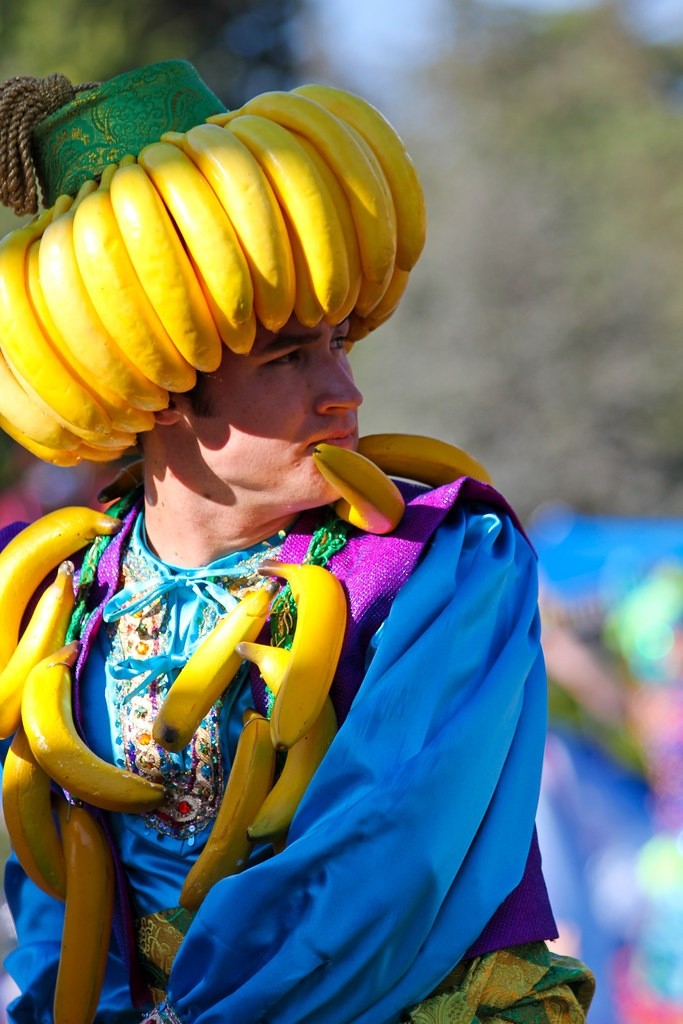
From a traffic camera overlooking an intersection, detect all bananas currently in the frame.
[0,81,427,467]
[0,433,495,1024]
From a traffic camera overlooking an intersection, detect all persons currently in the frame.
[525,570,683,1024]
[1,55,602,1024]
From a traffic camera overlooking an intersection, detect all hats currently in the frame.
[0,58,428,467]
